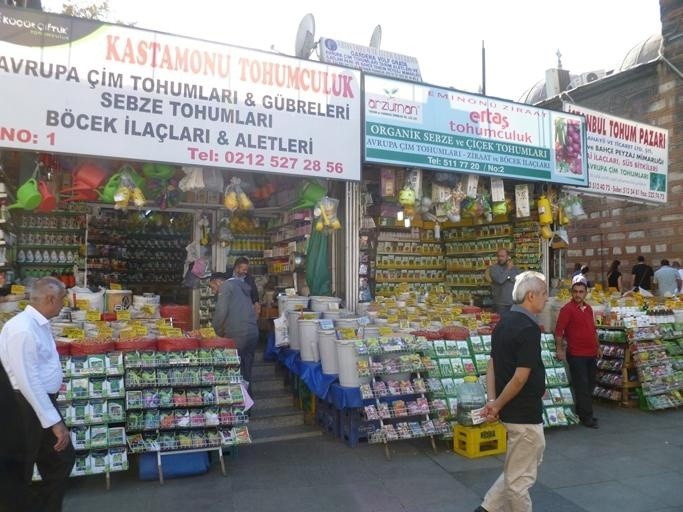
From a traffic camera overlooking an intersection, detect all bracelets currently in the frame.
[487,399,494,402]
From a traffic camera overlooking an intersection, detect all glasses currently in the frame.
[572,290,586,293]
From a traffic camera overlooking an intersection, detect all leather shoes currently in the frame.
[593,418,599,420]
[584,424,599,429]
[474,506,487,511]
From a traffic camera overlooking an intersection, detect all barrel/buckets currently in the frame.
[318,330,338,373]
[323,312,340,318]
[457,376,486,426]
[297,320,320,362]
[276,296,311,317]
[311,296,342,312]
[336,318,358,331]
[289,312,321,350]
[335,340,370,387]
[363,328,378,339]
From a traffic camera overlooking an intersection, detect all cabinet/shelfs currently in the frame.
[446,219,549,304]
[0,181,18,294]
[267,203,319,306]
[18,206,86,296]
[197,210,283,328]
[370,222,447,301]
[86,208,197,295]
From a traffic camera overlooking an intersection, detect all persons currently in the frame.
[654,260,682,299]
[572,267,590,288]
[475,272,548,511]
[569,264,583,278]
[211,274,259,415]
[490,250,517,316]
[607,261,622,295]
[0,278,75,511]
[632,256,653,298]
[555,282,601,427]
[671,261,682,299]
[229,257,261,322]
